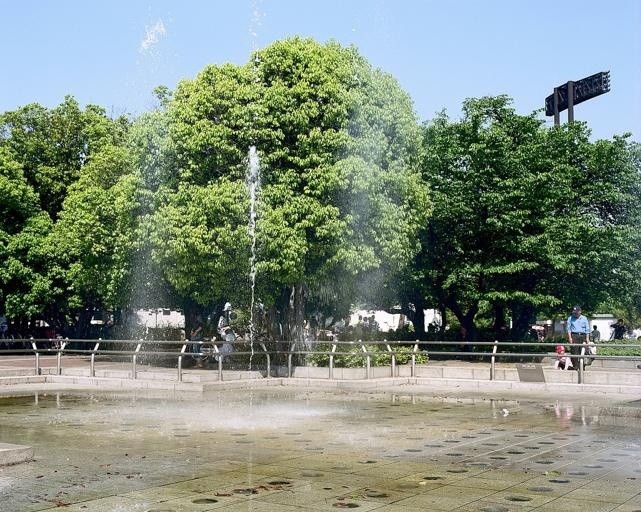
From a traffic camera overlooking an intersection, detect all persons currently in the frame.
[454,328,474,361]
[188,311,204,369]
[529,305,639,371]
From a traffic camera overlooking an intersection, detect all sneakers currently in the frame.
[568,366,578,370]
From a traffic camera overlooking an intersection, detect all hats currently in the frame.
[556,345,564,352]
[572,306,580,314]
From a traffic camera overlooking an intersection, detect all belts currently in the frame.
[572,332,585,334]
